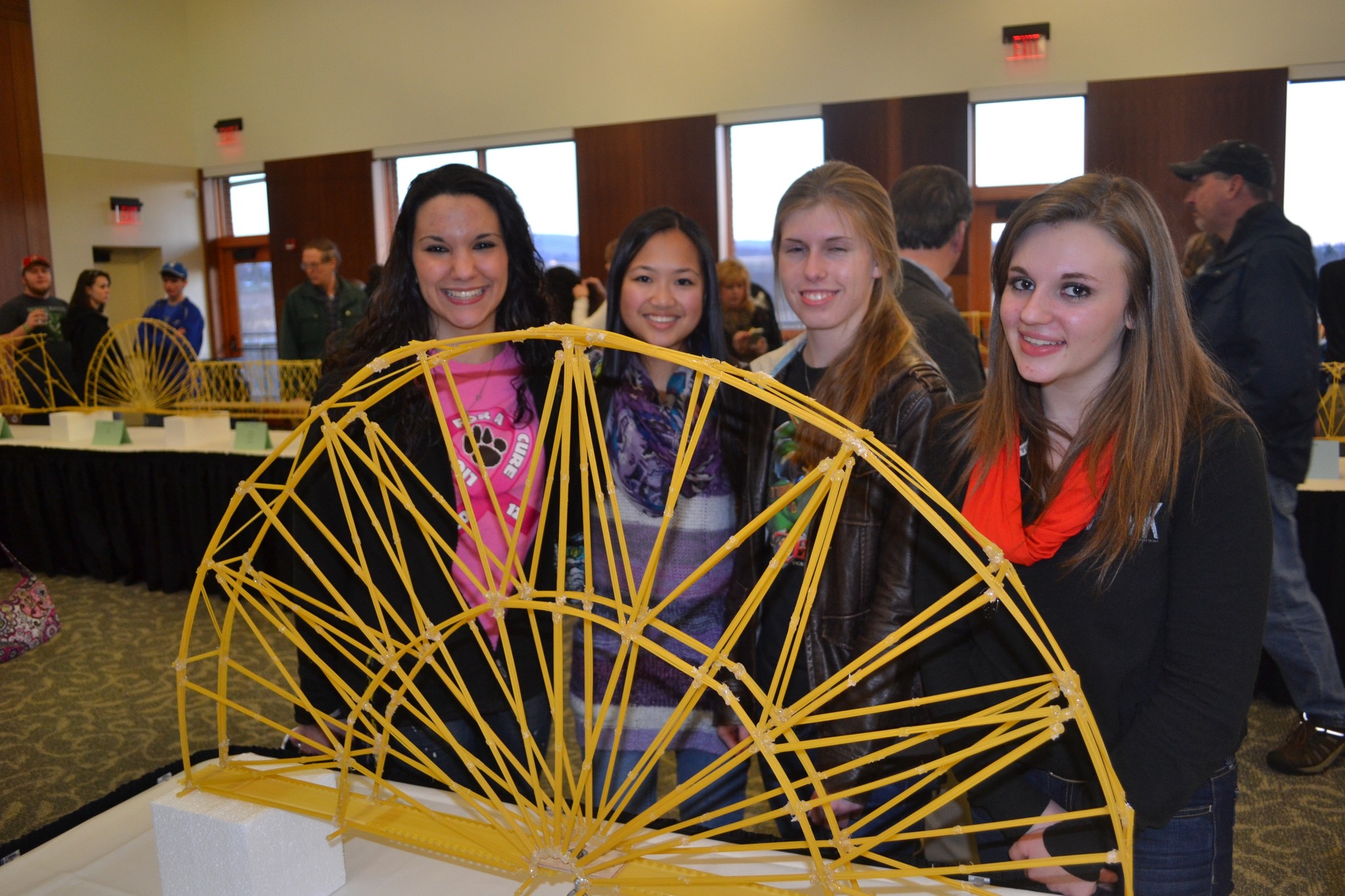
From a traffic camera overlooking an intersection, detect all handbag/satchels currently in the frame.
[0,542,61,665]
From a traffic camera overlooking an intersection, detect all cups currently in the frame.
[34,307,49,327]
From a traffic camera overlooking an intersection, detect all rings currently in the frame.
[37,320,42,324]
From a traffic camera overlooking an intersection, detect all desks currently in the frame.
[1,425,302,600]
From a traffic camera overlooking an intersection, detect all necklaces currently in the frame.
[1021,478,1046,502]
[465,346,498,414]
[804,347,814,395]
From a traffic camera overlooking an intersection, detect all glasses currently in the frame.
[300,260,329,270]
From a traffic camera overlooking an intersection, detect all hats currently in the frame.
[159,262,187,278]
[1168,139,1272,188]
[20,256,51,276]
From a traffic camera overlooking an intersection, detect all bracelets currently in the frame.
[21,322,29,335]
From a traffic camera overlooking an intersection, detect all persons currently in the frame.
[545,237,621,374]
[917,173,1273,896]
[1161,140,1345,776]
[132,259,205,406]
[285,237,385,411]
[888,165,987,410]
[712,259,782,367]
[711,161,958,867]
[284,164,600,838]
[556,206,754,838]
[0,255,69,425]
[58,269,128,410]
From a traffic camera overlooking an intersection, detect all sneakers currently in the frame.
[1269,714,1345,773]
